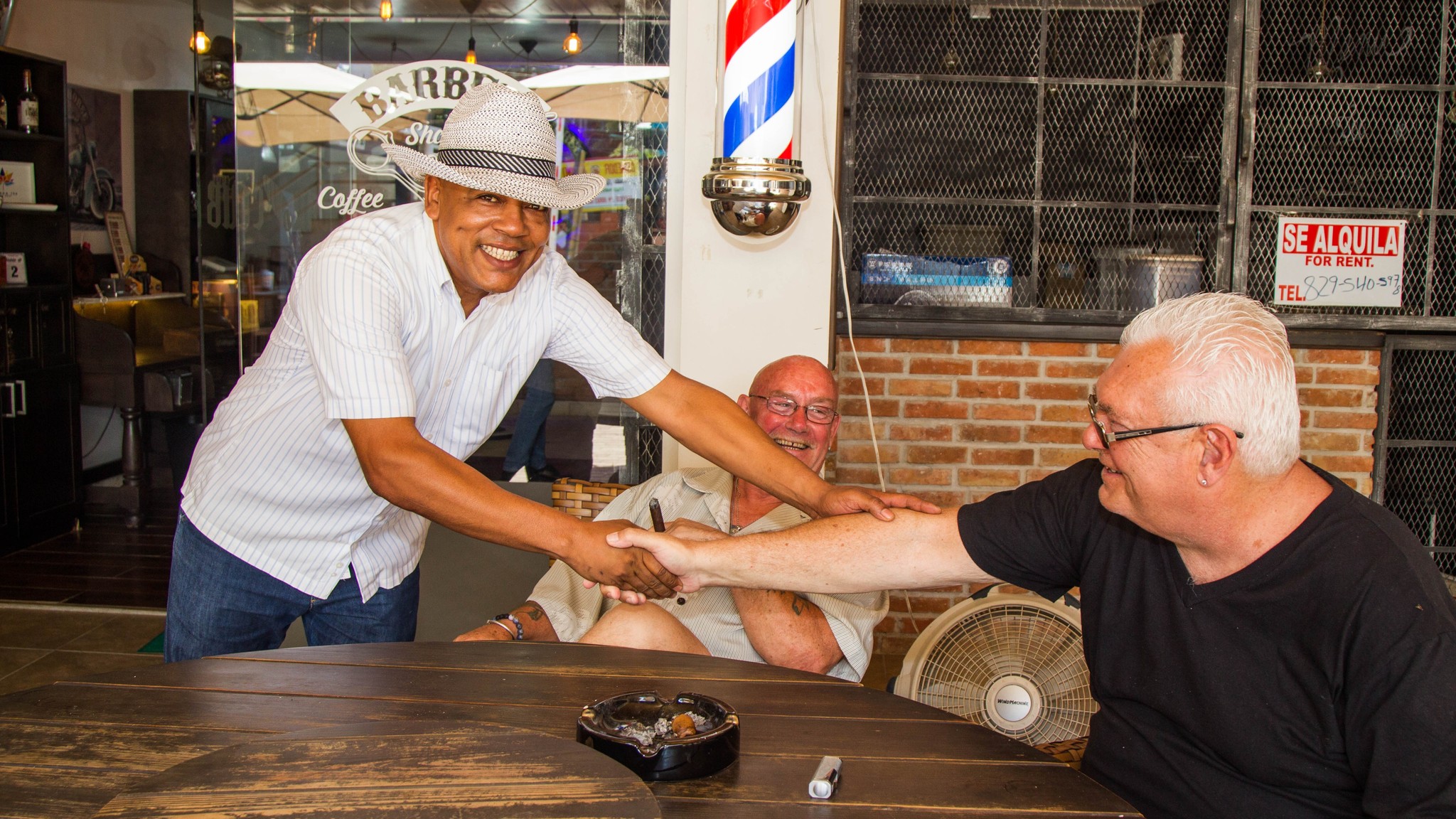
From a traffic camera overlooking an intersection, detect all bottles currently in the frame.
[0,93,7,129]
[17,69,38,132]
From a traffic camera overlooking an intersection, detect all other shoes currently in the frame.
[529,463,567,483]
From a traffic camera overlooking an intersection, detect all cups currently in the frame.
[258,272,274,291]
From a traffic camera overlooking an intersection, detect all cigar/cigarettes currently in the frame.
[649,498,665,533]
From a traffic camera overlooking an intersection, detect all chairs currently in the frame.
[551,474,632,564]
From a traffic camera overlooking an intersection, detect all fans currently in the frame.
[894,593,1096,748]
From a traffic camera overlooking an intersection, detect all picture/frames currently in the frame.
[0,161,36,204]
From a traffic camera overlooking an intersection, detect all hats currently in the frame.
[380,83,605,210]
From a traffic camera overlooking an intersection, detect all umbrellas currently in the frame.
[236,59,676,149]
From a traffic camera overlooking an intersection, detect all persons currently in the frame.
[455,355,889,683]
[581,294,1456,818]
[165,87,943,662]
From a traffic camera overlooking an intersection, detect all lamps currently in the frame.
[702,0,811,238]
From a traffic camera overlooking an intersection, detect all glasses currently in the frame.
[1087,393,1243,450]
[749,394,838,424]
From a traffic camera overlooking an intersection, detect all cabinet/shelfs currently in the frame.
[0,46,76,561]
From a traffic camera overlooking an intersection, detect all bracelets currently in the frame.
[487,612,522,641]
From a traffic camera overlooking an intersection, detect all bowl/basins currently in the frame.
[924,224,1005,258]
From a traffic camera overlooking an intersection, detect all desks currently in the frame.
[0,643,1148,819]
[73,289,238,527]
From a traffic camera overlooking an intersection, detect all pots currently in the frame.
[1095,254,1205,312]
